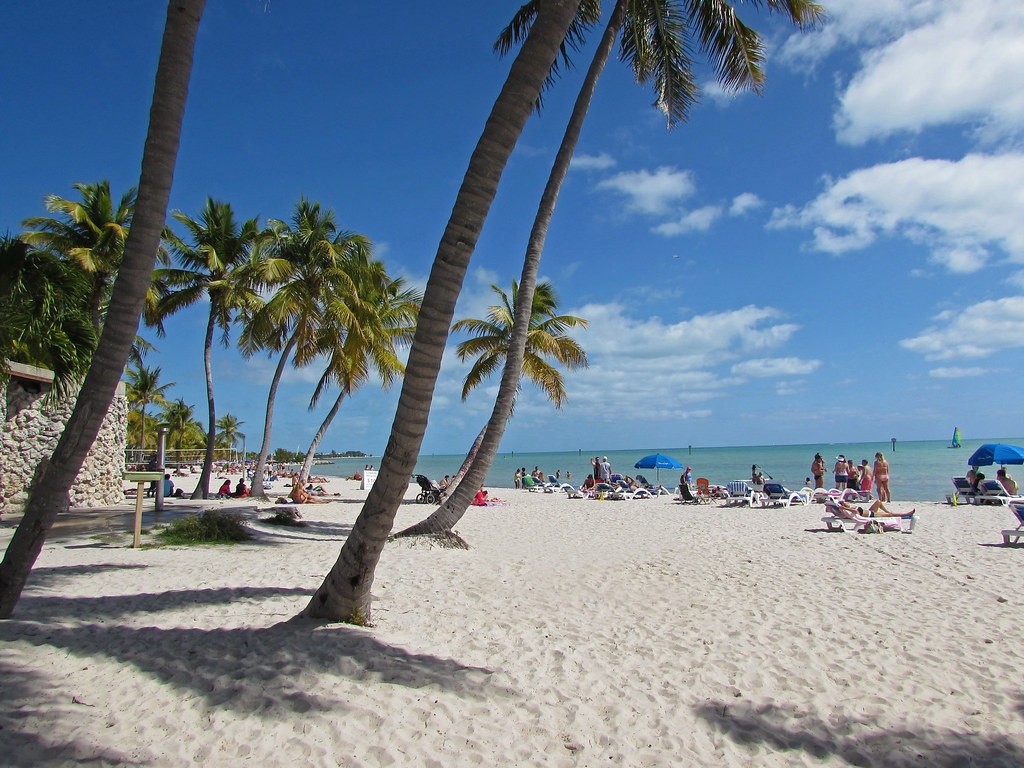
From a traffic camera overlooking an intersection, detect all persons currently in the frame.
[582,456,661,491]
[996,470,1017,495]
[811,453,825,488]
[806,477,811,485]
[838,500,915,517]
[355,464,374,480]
[440,474,455,488]
[566,471,572,479]
[967,466,985,495]
[682,466,692,485]
[513,466,544,489]
[164,474,174,497]
[832,451,891,502]
[471,484,489,506]
[752,465,765,484]
[189,464,329,504]
[556,470,561,478]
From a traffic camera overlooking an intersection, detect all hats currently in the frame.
[686,466,692,470]
[603,456,608,461]
[835,455,845,462]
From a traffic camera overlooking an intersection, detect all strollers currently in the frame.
[411,473,440,506]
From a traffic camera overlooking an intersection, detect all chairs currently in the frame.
[522,474,920,534]
[945,476,1024,544]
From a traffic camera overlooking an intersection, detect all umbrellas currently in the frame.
[634,452,682,481]
[968,443,1024,469]
